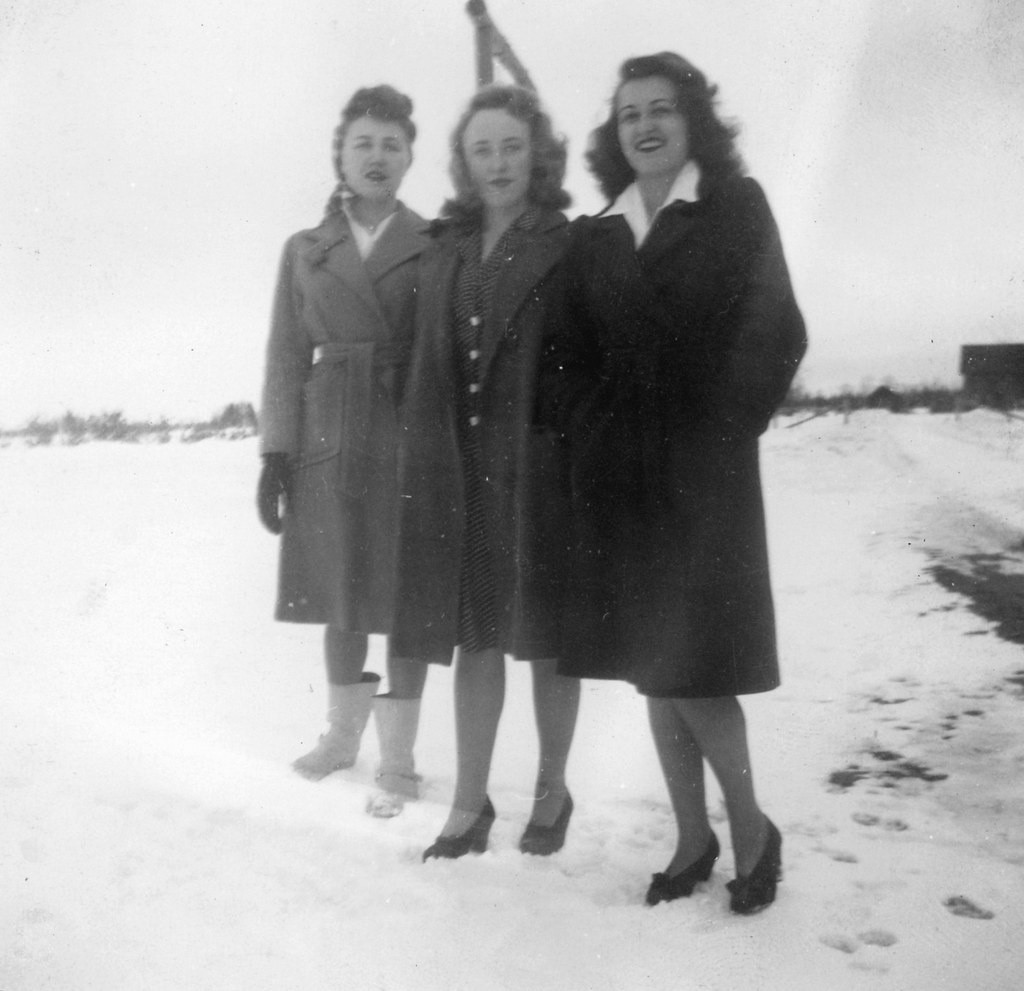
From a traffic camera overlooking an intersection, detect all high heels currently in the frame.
[646,837,719,911]
[426,796,494,863]
[516,798,577,855]
[727,820,782,915]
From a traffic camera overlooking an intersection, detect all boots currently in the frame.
[293,674,378,779]
[368,694,421,818]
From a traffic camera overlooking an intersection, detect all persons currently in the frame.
[388,82,576,863]
[539,50,810,915]
[255,75,438,811]
